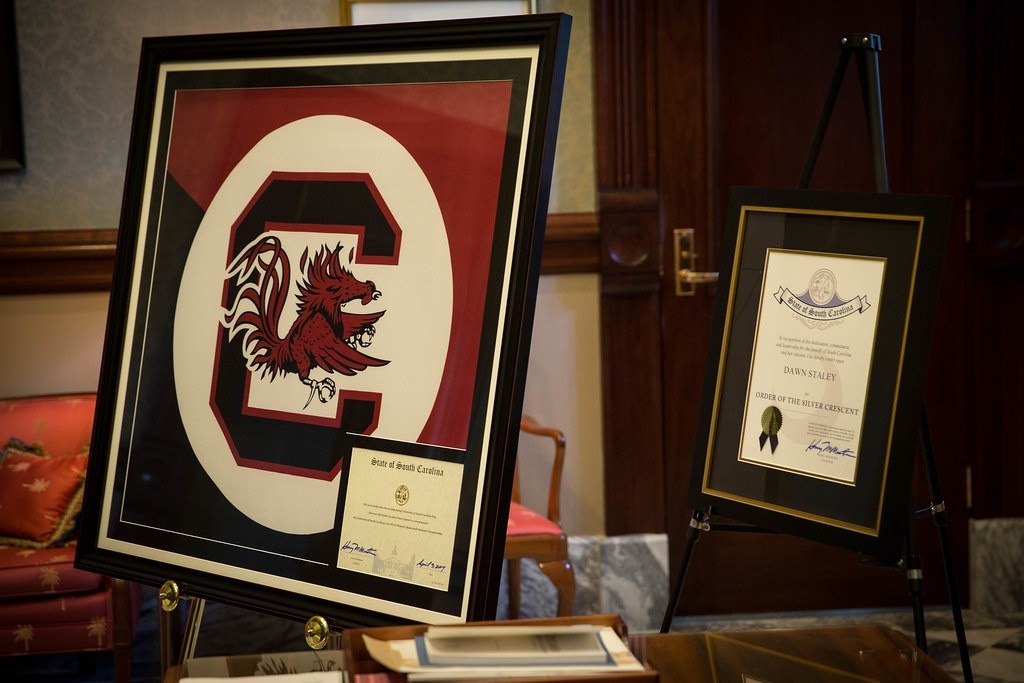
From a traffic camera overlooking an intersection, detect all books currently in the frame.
[364,622,648,683]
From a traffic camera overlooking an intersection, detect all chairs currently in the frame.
[493,420,570,621]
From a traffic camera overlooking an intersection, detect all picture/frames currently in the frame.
[73,12,571,624]
[702,207,925,541]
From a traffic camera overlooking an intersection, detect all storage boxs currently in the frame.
[968,520,1023,611]
[495,533,670,633]
[339,612,659,683]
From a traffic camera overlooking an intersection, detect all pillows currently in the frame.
[0,398,96,549]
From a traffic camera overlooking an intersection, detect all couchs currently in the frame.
[0,387,146,683]
